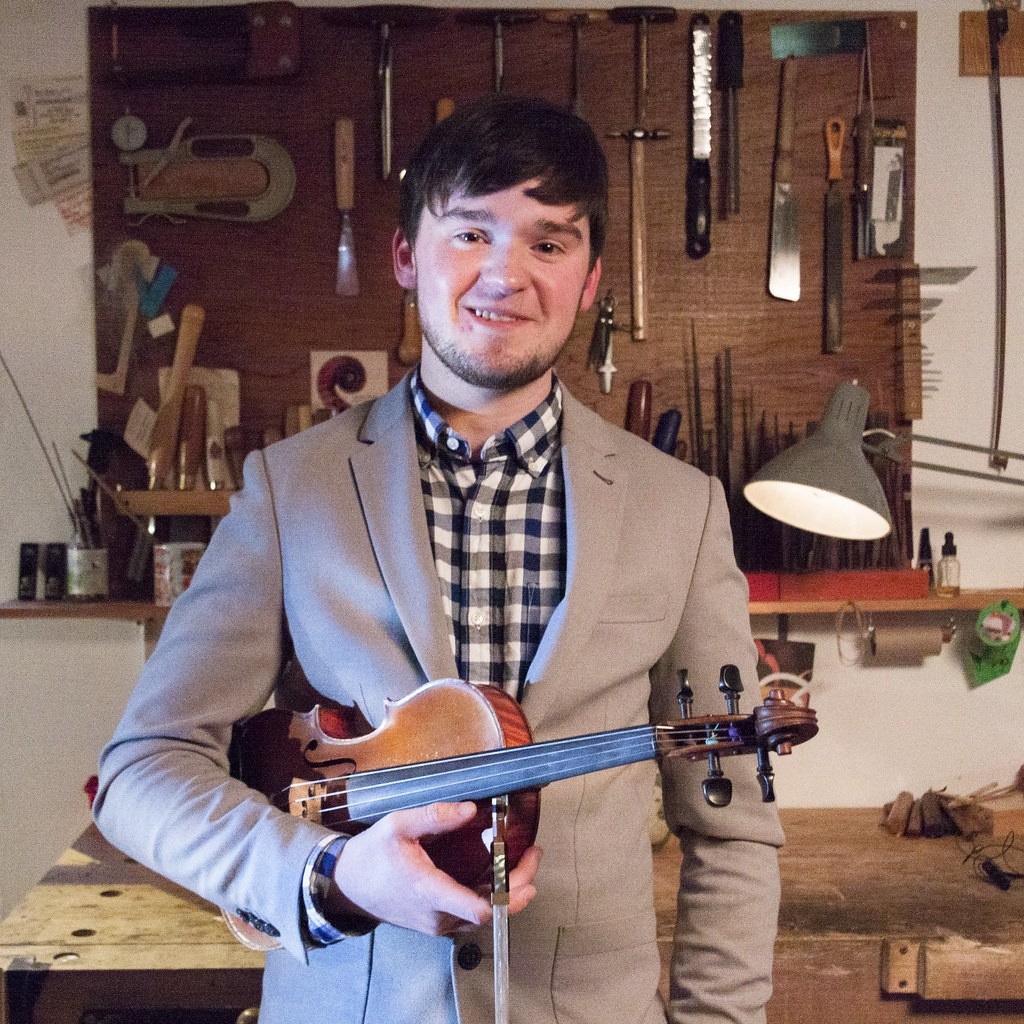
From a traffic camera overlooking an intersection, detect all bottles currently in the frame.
[936,532,960,598]
[67,499,109,602]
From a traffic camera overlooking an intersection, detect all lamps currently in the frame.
[742,378,1024,540]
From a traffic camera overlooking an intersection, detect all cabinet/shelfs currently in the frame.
[2,487,1024,663]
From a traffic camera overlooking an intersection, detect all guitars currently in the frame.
[222,661,820,951]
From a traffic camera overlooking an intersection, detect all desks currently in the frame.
[0,801,1024,1024]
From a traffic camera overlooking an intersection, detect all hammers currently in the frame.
[608,5,679,121]
[607,128,671,345]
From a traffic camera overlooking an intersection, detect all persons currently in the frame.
[88,92,798,1024]
